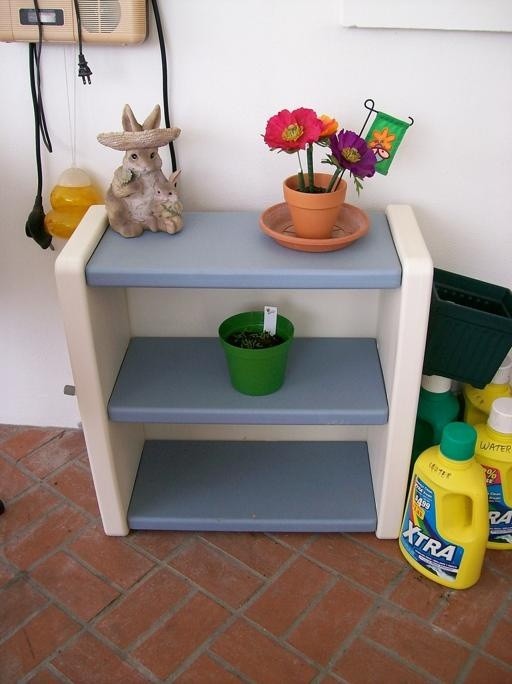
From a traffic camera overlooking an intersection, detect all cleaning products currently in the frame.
[476,397,512,553]
[413,374,460,462]
[462,358,511,424]
[394,419,491,591]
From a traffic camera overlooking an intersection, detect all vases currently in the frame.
[217,310,294,398]
[282,172,348,239]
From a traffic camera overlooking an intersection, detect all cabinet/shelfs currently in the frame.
[52,203,435,540]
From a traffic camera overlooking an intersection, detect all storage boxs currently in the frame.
[423,267,511,390]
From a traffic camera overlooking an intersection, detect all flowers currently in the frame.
[258,105,378,199]
